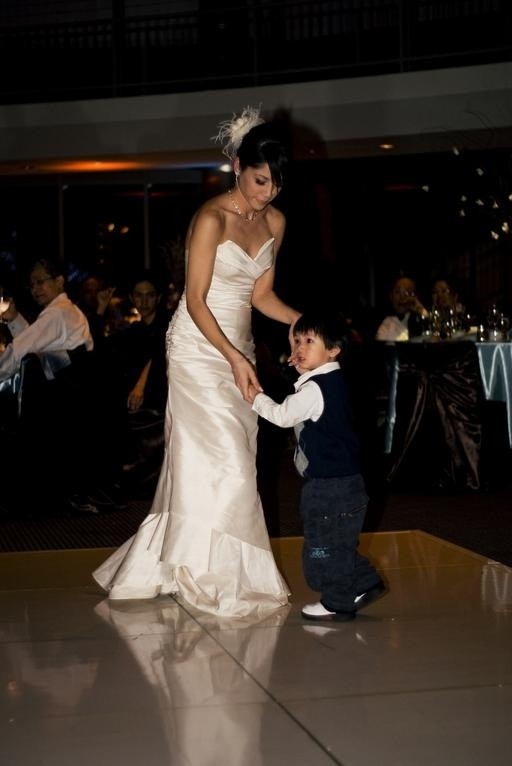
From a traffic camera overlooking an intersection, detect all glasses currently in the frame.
[28,276,59,289]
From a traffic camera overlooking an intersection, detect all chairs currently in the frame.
[391,340,495,498]
[17,353,48,418]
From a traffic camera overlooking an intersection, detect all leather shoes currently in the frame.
[300,600,357,623]
[356,582,384,609]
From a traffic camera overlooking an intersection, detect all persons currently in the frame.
[371,270,476,341]
[246,308,390,620]
[81,268,218,472]
[0,255,96,438]
[93,105,305,618]
